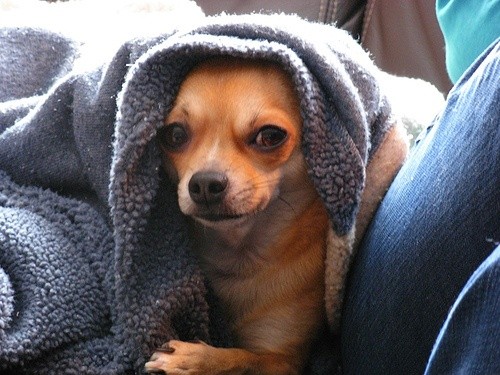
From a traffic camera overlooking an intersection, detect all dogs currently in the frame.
[143,59,408,375]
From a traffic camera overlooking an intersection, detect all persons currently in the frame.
[336,1,500,375]
